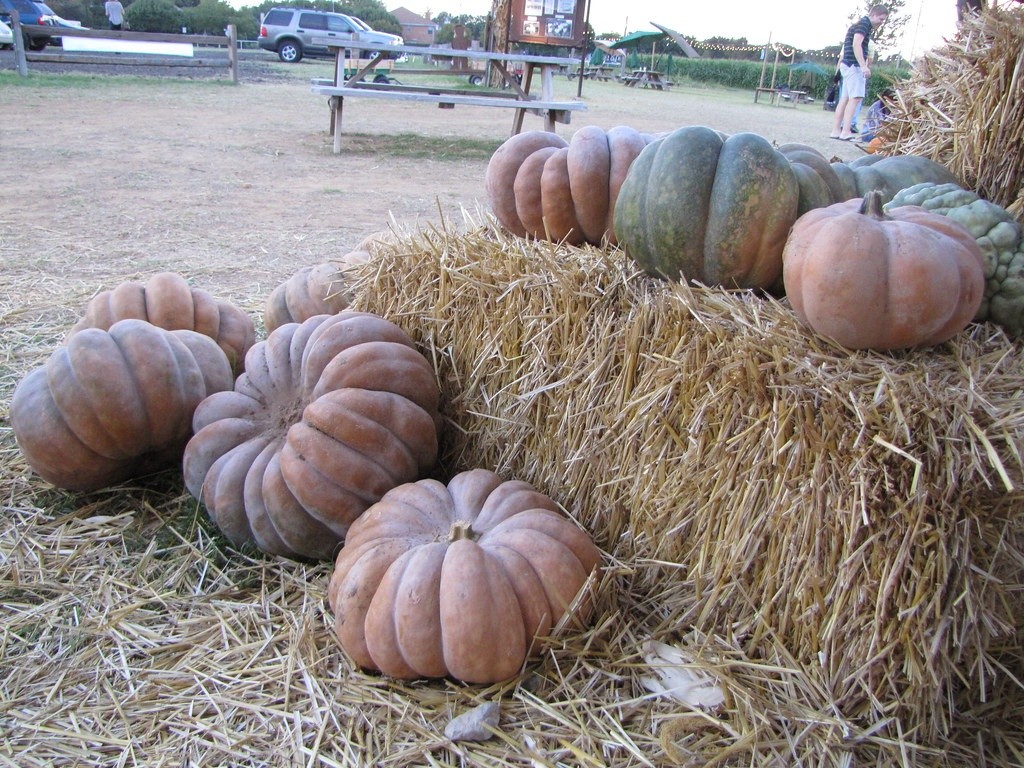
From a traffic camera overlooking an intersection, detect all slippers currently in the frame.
[830,135,840,139]
[838,134,855,141]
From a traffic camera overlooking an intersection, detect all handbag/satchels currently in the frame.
[823,83,839,112]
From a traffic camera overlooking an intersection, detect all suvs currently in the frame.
[257,5,404,63]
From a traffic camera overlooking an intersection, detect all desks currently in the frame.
[311,37,582,154]
[572,67,614,82]
[624,70,671,92]
[754,87,799,109]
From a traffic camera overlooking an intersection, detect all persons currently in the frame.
[823,4,898,154]
[104,0,125,55]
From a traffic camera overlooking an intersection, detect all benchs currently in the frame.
[309,77,586,110]
[570,72,614,79]
[621,76,674,86]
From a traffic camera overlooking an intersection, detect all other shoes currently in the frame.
[850,125,860,132]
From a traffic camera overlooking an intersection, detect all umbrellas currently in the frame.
[589,21,700,88]
[786,61,830,85]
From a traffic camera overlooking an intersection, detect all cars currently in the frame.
[0,0,58,51]
[351,16,374,31]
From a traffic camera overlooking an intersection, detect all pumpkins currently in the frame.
[329,469,605,684]
[484,122,1024,352]
[7,319,235,491]
[59,272,255,381]
[263,233,400,336]
[183,310,439,559]
